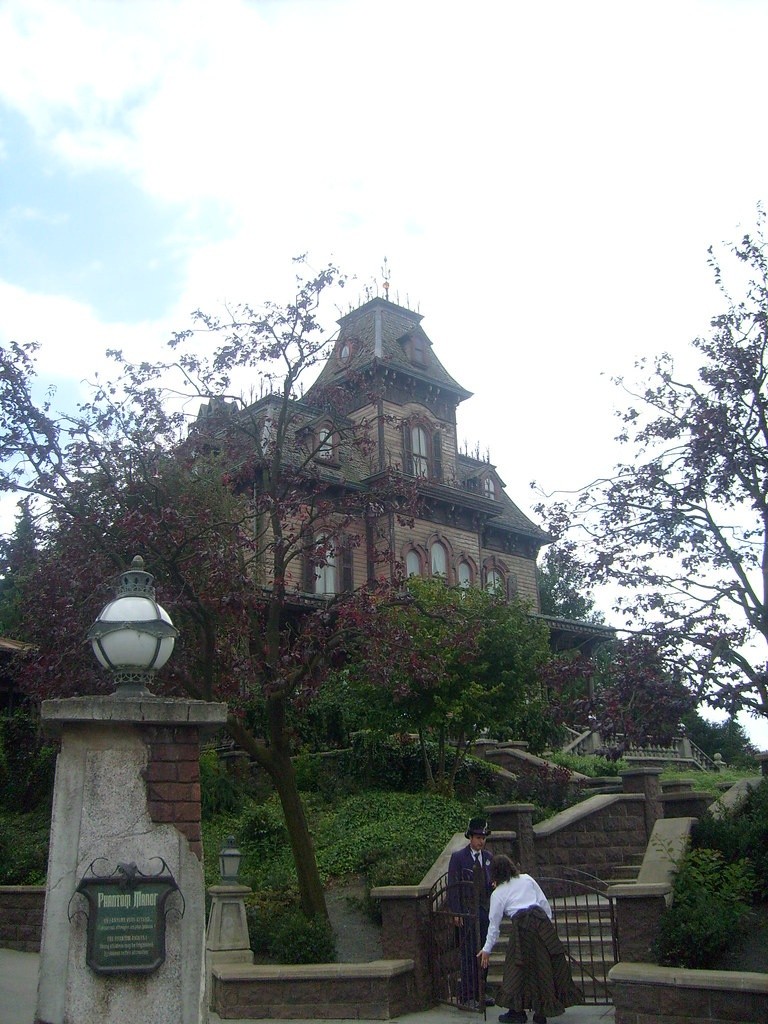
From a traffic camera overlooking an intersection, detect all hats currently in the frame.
[464,817,491,839]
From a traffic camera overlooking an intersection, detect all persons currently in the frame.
[447,818,499,1009]
[476,853,583,1024]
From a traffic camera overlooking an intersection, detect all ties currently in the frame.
[469,848,489,912]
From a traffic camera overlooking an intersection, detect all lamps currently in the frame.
[81,555,181,698]
[676,721,686,736]
[215,835,244,884]
[473,722,491,739]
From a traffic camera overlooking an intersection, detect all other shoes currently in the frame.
[533,1013,547,1023]
[458,1000,480,1011]
[498,1009,528,1024]
[478,995,495,1006]
